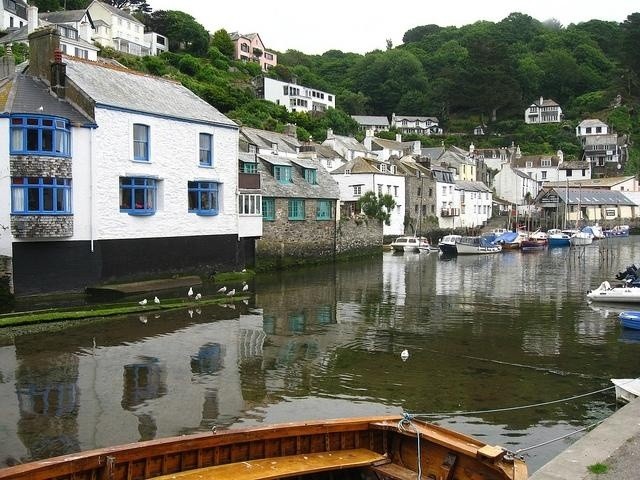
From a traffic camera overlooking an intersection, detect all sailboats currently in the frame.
[438,173,605,261]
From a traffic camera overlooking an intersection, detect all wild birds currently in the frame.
[154,296,160,304]
[242,284,248,293]
[216,286,226,294]
[188,287,194,297]
[227,288,236,298]
[400,349,409,361]
[138,299,147,306]
[195,293,201,301]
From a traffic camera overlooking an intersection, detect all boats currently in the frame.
[585,301,640,319]
[583,280,640,304]
[2,408,534,480]
[615,310,640,331]
[388,234,432,253]
[392,252,421,265]
[603,224,630,238]
[418,247,441,254]
